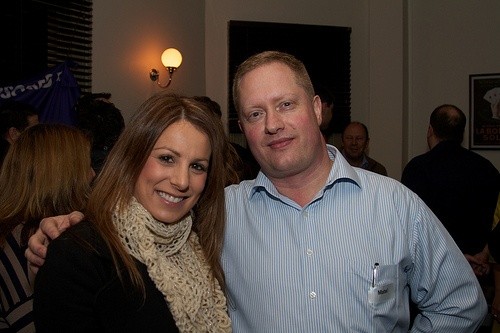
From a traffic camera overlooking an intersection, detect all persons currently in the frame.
[24,50,488,333]
[33,94,237,333]
[312,74,388,178]
[401,105,500,333]
[0,105,95,333]
[193,96,261,183]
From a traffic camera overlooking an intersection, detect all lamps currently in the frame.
[150,48,182,89]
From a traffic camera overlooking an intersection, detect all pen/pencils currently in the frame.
[372,263,380,288]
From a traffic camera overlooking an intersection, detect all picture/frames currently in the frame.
[469,73,500,151]
